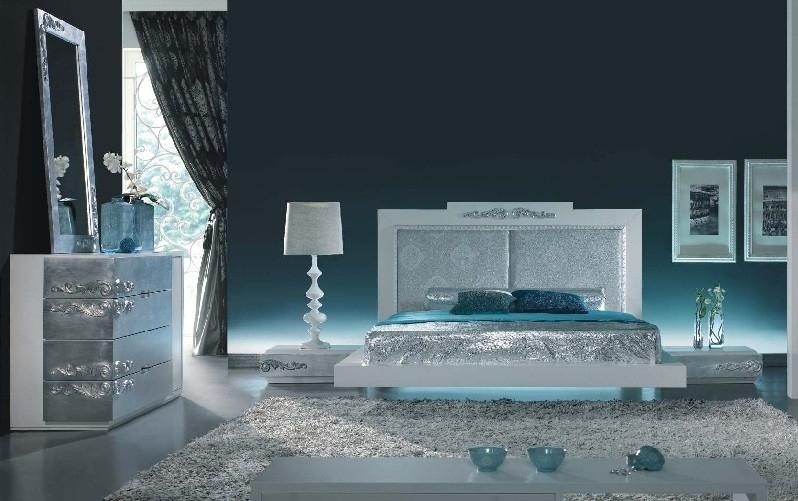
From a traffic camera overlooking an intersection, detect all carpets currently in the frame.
[98,396,798,501]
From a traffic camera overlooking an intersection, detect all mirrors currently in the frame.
[34,7,99,254]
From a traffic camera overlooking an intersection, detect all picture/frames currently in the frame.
[671,159,737,264]
[744,158,789,262]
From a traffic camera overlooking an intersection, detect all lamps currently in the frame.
[283,201,344,349]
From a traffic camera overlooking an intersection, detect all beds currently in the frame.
[335,286,688,408]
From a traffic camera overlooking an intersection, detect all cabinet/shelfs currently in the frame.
[8,252,186,431]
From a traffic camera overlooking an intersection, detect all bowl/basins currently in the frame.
[469,446,508,472]
[527,446,565,472]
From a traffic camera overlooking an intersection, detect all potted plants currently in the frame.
[693,286,712,350]
[708,282,728,350]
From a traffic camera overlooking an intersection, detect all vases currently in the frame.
[130,198,156,252]
[102,197,136,253]
[59,201,77,236]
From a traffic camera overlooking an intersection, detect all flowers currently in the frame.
[53,153,71,203]
[104,152,176,211]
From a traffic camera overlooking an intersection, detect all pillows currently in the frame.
[513,291,586,298]
[452,289,503,308]
